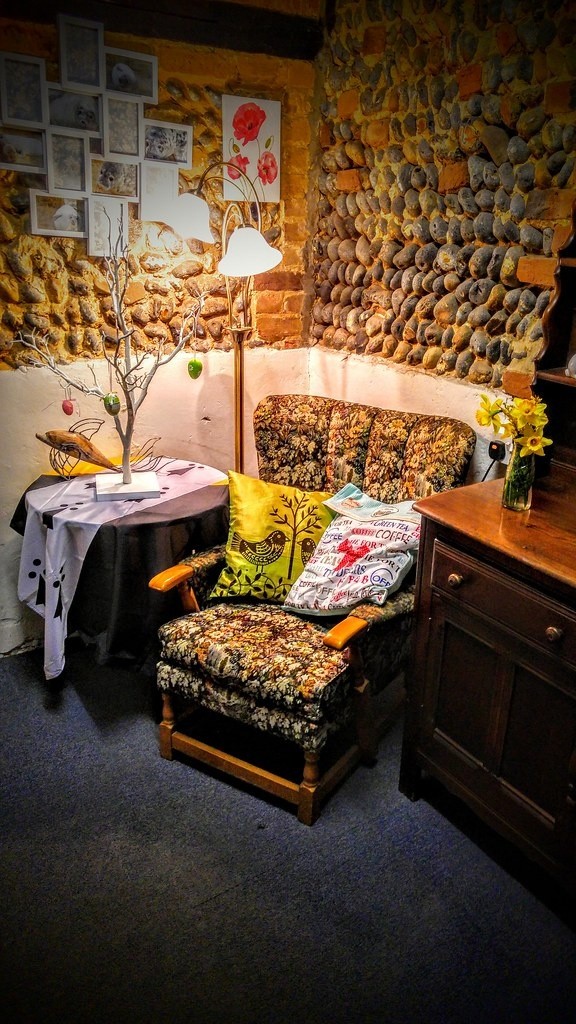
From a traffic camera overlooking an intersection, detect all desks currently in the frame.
[10,458,228,680]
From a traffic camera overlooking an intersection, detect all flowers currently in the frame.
[477,395,553,457]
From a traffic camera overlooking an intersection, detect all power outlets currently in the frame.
[499,443,513,465]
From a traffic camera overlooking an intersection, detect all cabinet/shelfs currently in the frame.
[396,193,576,922]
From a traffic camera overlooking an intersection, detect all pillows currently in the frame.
[283,483,421,616]
[209,470,335,601]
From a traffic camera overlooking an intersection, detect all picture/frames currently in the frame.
[0,15,192,256]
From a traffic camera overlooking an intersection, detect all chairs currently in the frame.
[148,394,476,826]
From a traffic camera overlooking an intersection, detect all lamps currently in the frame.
[152,162,283,475]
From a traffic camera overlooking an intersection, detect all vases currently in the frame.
[502,440,537,511]
